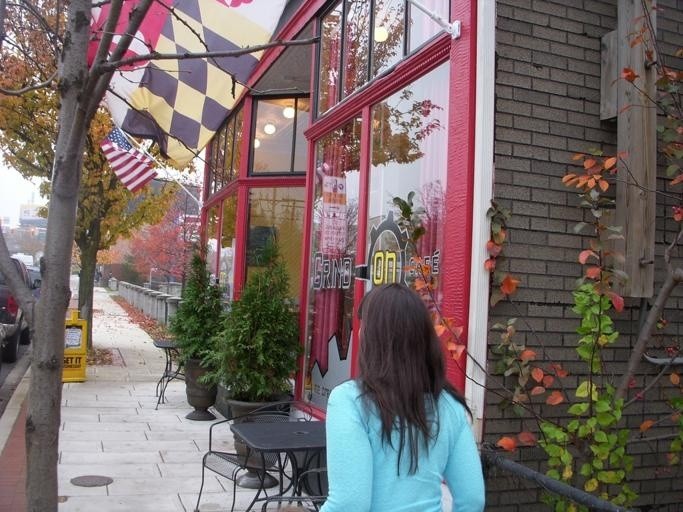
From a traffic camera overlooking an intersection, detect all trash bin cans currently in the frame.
[111,279,118,291]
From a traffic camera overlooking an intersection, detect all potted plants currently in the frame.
[166,223,302,428]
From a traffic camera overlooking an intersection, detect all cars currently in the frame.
[0,254,39,365]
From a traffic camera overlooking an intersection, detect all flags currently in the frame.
[98,127,158,193]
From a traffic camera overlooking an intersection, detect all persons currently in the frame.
[311,283,487,510]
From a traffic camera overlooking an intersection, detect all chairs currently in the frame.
[150,338,192,412]
[193,400,328,511]
